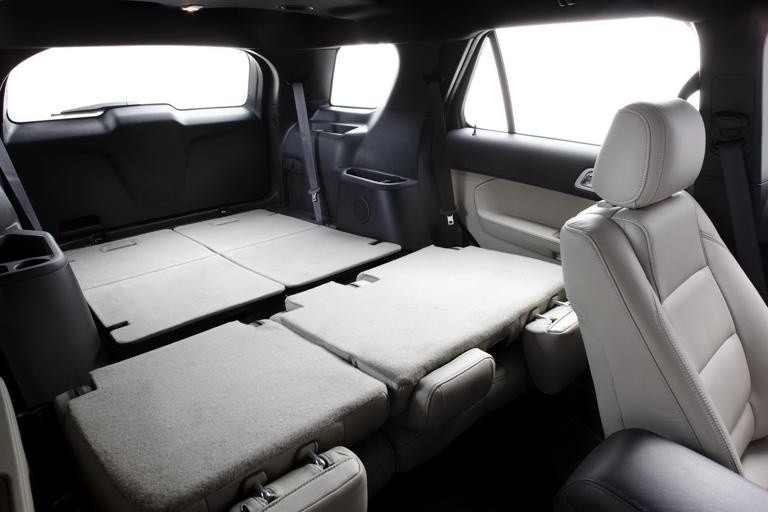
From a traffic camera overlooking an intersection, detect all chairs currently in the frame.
[559,98,768,512]
